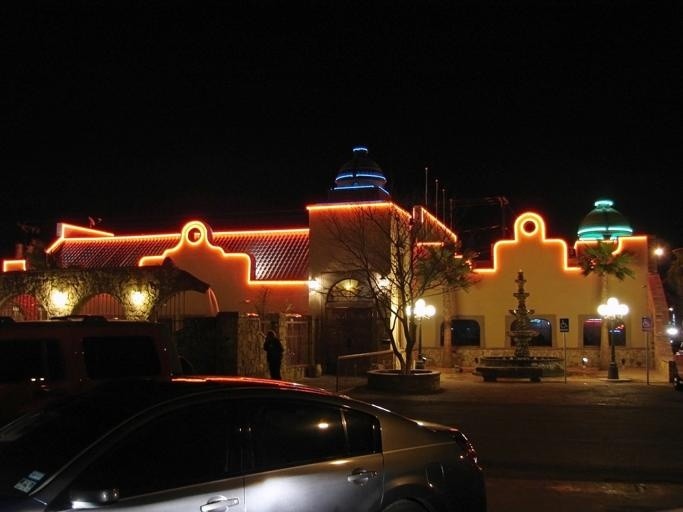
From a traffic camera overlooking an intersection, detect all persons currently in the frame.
[261,330,286,379]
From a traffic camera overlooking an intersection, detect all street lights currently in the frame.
[406,300,437,371]
[598,297,631,380]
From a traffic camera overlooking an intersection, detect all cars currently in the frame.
[1,375,488,512]
[674,340,683,392]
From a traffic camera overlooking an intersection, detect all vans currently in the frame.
[0,317,180,419]
